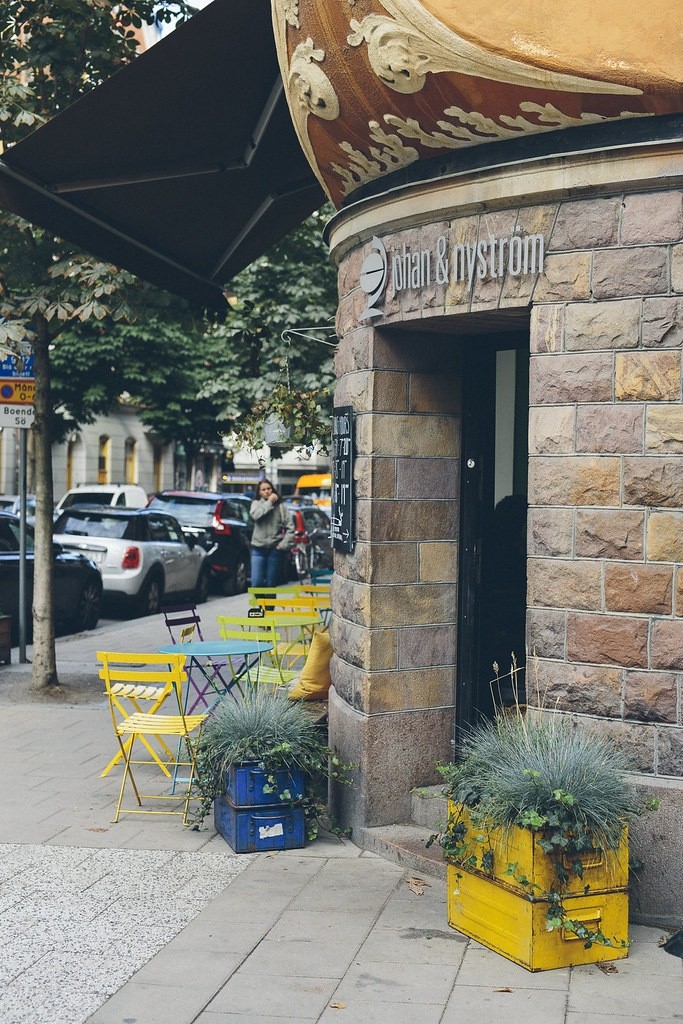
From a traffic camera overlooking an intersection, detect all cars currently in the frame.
[57,481,149,518]
[0,493,48,526]
[280,473,331,540]
[49,505,206,617]
[0,510,104,653]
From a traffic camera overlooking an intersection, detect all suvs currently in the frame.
[142,490,264,592]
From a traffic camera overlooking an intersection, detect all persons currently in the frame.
[247,478,296,611]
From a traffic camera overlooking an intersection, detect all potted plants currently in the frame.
[184,696,359,855]
[434,703,638,976]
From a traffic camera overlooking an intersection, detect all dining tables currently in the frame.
[156,639,276,781]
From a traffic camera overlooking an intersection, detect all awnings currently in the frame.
[0,0,330,310]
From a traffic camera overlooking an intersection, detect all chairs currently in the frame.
[217,569,331,698]
[93,650,207,826]
[162,602,217,676]
